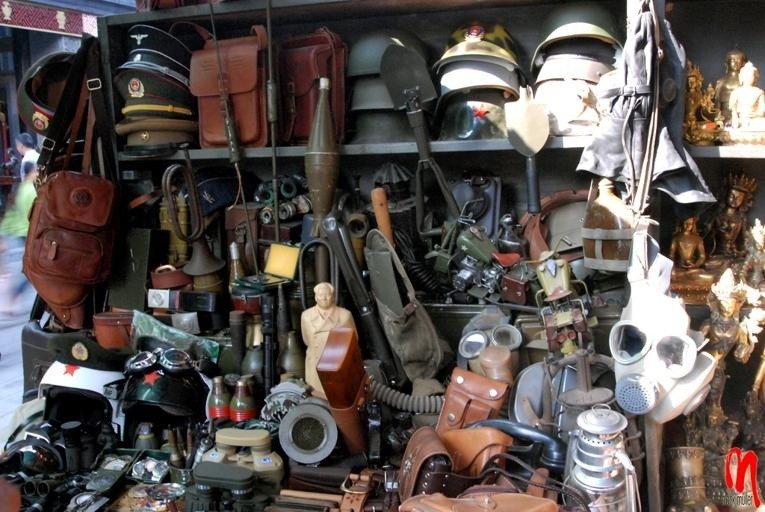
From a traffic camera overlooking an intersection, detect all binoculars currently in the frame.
[24,472,67,498]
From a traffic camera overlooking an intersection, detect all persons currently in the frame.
[665,171,765,489]
[688,46,765,132]
[0,133,40,308]
[300,282,359,400]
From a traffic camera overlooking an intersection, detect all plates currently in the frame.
[279,404,338,464]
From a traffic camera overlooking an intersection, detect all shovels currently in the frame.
[380,45,460,237]
[504,101,550,213]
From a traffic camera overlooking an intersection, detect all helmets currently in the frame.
[348,3,622,142]
[1,349,214,473]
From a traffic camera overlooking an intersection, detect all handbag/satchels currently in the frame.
[400,372,560,511]
[193,30,346,149]
[22,170,117,402]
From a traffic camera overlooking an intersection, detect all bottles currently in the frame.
[205,310,303,424]
[224,242,245,293]
[136,419,156,451]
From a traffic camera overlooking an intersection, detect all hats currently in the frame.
[23,25,196,156]
